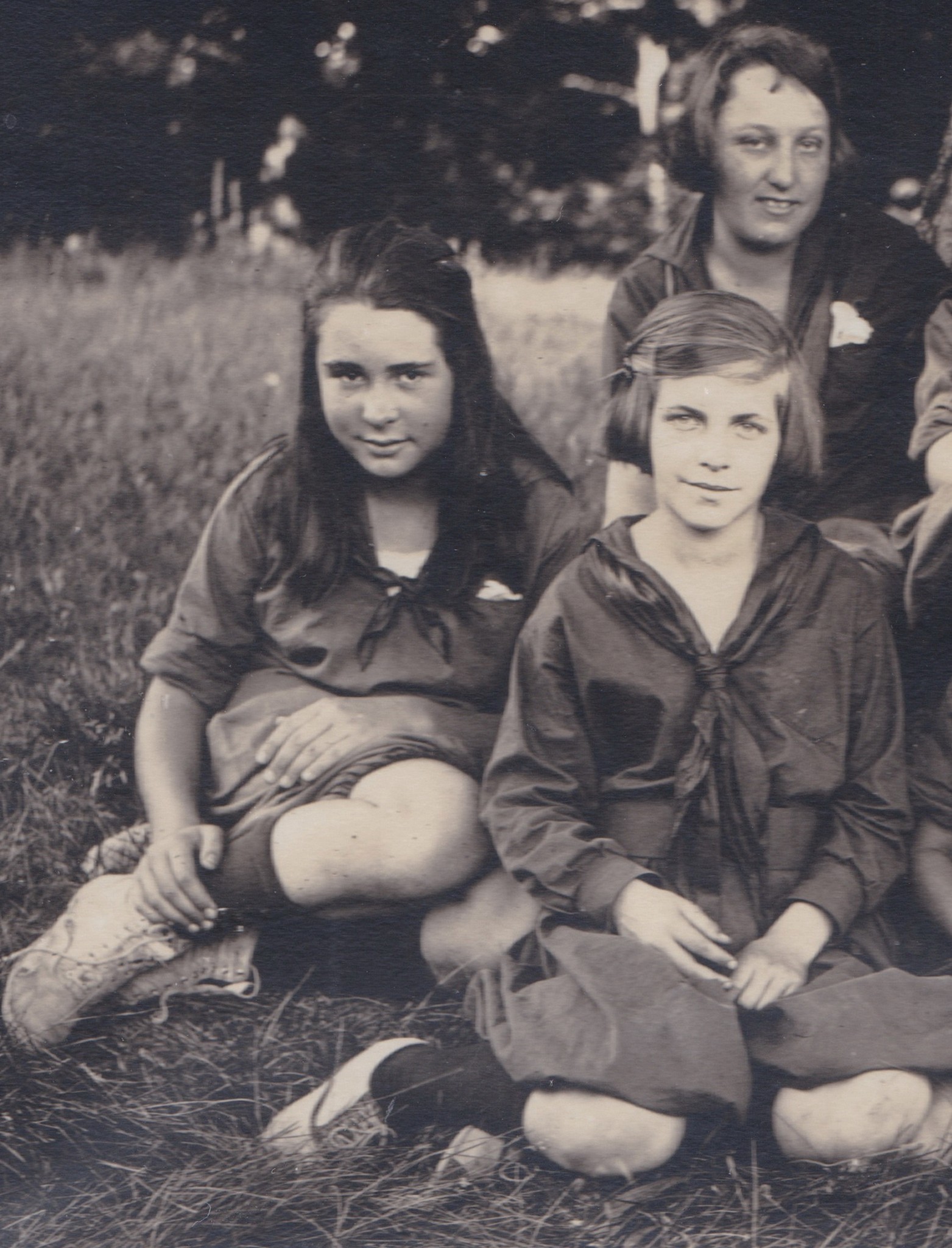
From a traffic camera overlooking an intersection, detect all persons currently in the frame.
[910,754,952,936]
[907,299,952,489]
[598,22,952,618]
[1,219,598,1054]
[259,290,935,1183]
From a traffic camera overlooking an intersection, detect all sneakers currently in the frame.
[429,1124,532,1183]
[258,1038,436,1167]
[2,873,196,1056]
[106,919,262,1002]
